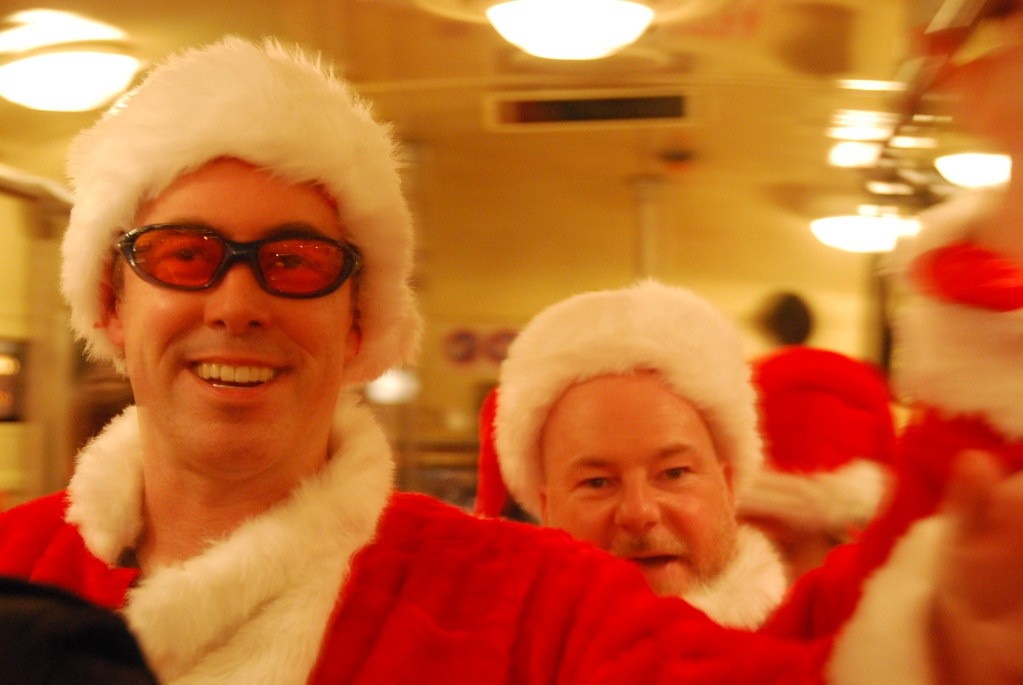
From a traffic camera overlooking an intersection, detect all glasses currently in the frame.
[113,222,363,299]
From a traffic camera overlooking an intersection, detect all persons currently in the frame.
[752,241,1022,635]
[496,290,805,636]
[0,38,1023,685]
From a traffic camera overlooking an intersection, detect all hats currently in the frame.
[889,242,1023,441]
[491,279,764,523]
[60,37,423,386]
[737,347,894,535]
